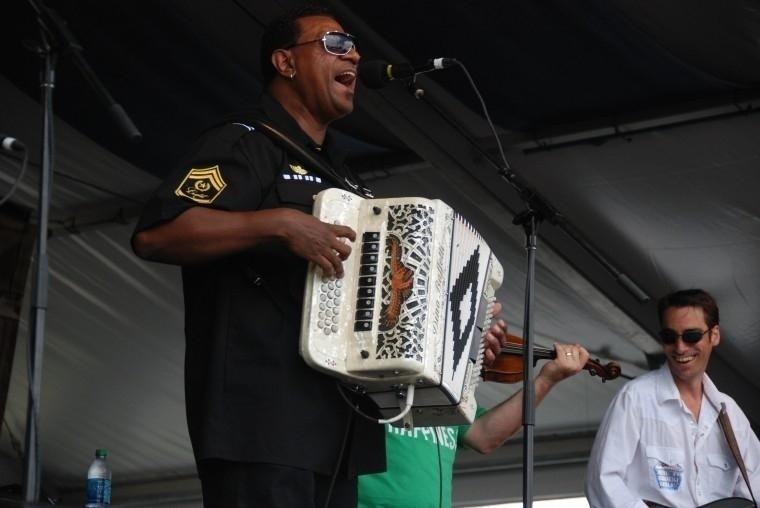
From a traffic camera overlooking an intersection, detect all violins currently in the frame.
[480,335,621,383]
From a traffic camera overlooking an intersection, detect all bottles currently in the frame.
[84,447,113,508]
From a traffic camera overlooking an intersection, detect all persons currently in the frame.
[358,341,591,506]
[132,9,510,507]
[584,288,760,505]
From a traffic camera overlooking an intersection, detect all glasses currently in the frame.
[291,32,358,55]
[660,326,709,342]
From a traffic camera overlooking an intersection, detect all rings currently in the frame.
[565,351,573,356]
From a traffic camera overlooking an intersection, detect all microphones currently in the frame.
[358,57,454,92]
